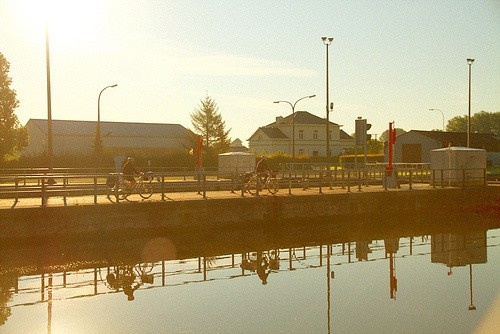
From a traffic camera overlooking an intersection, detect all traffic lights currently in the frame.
[355,120,374,148]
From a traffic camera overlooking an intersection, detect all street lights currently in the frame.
[95,84,117,143]
[467,265,476,311]
[321,36,335,176]
[429,107,446,130]
[272,94,317,164]
[464,58,475,148]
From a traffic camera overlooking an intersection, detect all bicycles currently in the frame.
[247,249,280,272]
[113,262,155,289]
[246,169,280,195]
[114,173,154,200]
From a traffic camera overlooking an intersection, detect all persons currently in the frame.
[122,157,145,193]
[256,156,271,189]
